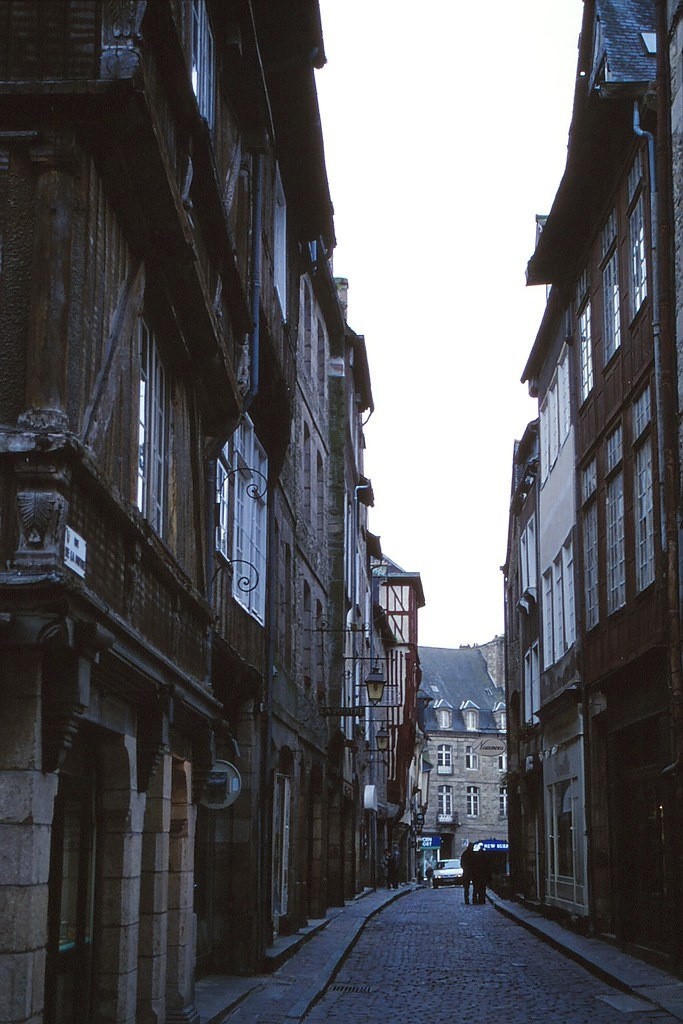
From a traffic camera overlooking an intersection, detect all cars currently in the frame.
[433,859,465,889]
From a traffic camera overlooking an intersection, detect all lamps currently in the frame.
[360,720,393,753]
[330,656,389,707]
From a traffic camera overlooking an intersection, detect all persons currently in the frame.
[416,862,433,884]
[460,842,491,904]
[380,843,400,889]
